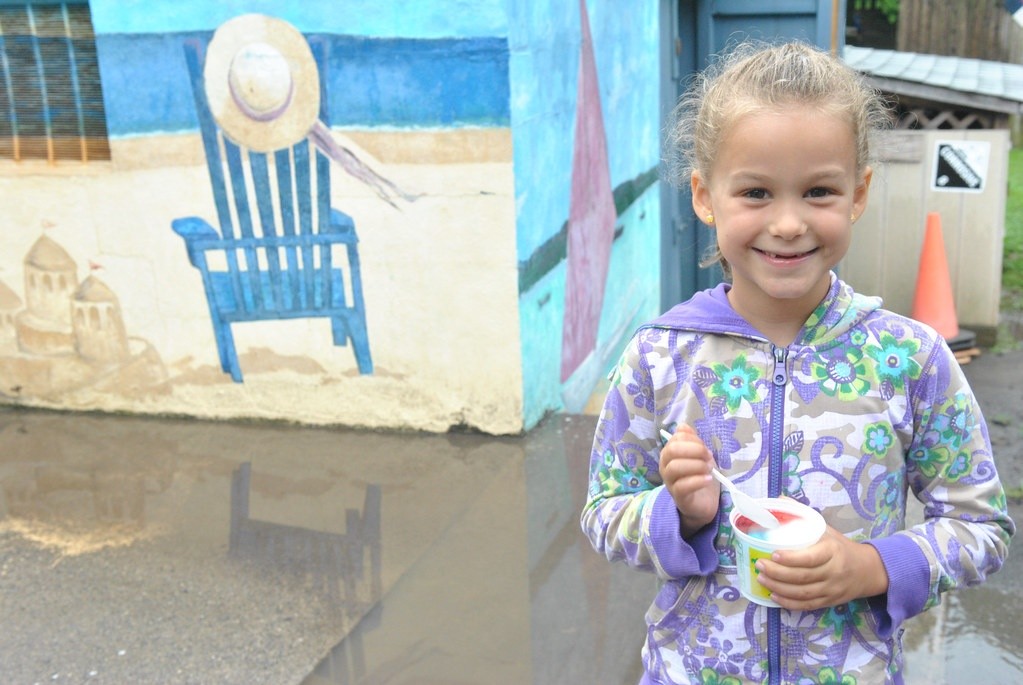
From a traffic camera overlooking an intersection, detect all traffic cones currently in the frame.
[903,211,977,352]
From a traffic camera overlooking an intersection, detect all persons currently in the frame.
[578,44,1016,685]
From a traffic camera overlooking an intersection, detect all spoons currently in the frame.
[656,427,777,527]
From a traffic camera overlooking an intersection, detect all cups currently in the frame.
[729,498,826,608]
